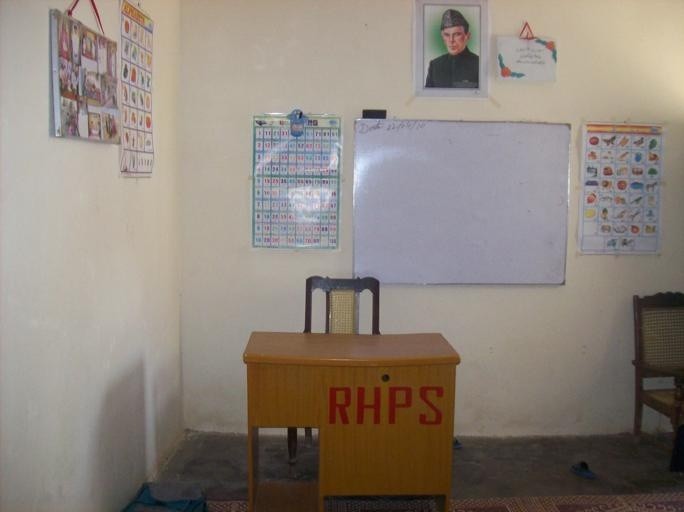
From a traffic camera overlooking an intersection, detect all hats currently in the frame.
[440,9,468,29]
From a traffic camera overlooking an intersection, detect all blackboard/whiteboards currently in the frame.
[352,119,571,285]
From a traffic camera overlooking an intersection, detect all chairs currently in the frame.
[287,275,382,463]
[632,290,682,473]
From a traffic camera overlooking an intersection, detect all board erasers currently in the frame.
[363,110,387,118]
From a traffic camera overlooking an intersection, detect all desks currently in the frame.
[242,330,461,509]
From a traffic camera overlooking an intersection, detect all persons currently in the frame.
[425,8,478,88]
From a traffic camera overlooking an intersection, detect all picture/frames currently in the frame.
[410,1,490,101]
